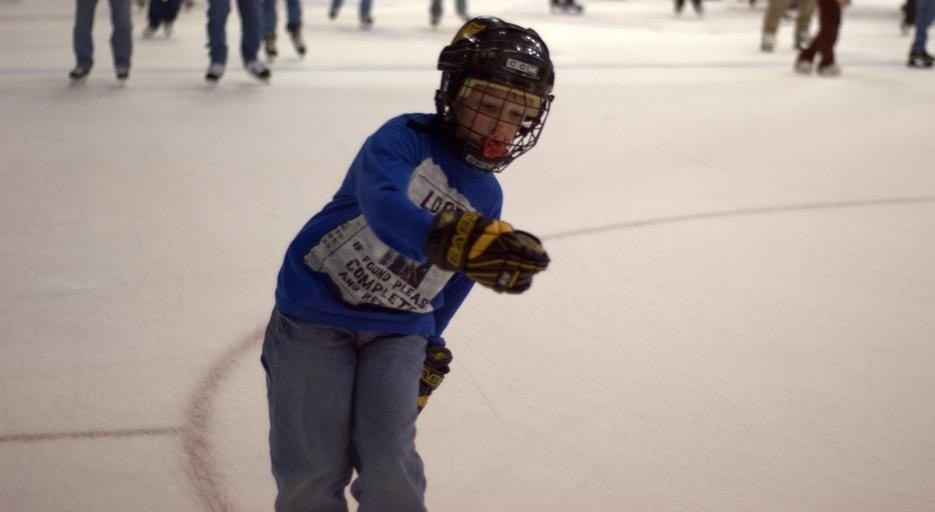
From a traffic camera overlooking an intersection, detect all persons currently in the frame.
[429,0,471,23]
[327,0,373,24]
[262,14,552,511]
[139,0,195,40]
[68,0,131,78]
[550,0,583,15]
[263,0,305,53]
[205,0,271,80]
[674,1,704,14]
[749,1,935,75]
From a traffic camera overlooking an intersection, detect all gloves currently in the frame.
[418,345,452,420]
[429,205,549,295]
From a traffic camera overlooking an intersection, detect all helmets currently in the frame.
[435,15,555,168]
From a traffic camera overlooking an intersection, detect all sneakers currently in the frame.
[669,2,935,83]
[69,31,309,87]
